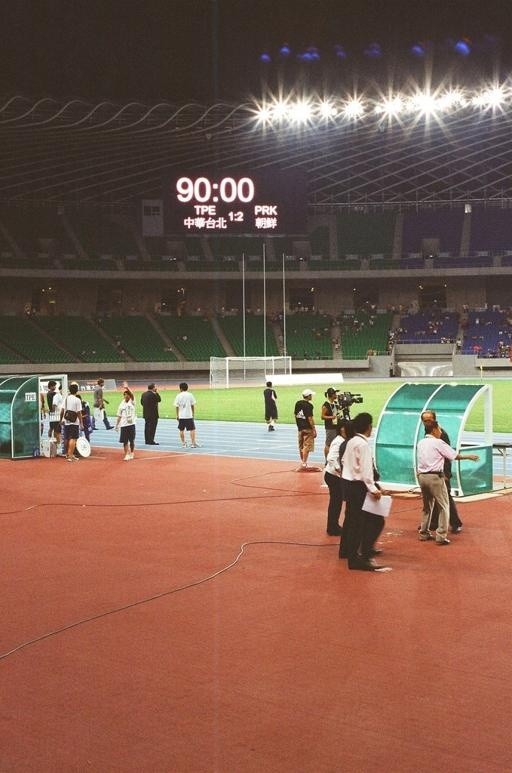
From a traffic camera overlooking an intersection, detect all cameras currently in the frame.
[337,392,363,418]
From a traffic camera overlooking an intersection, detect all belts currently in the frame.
[419,471,440,474]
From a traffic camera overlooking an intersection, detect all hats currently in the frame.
[301,389,316,397]
[327,387,341,394]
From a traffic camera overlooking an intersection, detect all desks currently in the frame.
[461,441,512,489]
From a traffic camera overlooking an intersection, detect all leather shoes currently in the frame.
[147,442,159,445]
[145,440,149,444]
[92,427,100,430]
[338,551,362,558]
[452,524,463,534]
[327,528,343,535]
[364,548,384,555]
[419,534,433,541]
[107,426,115,430]
[437,538,451,544]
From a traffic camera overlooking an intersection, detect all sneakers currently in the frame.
[190,444,202,449]
[129,453,134,460]
[123,454,130,460]
[182,443,187,449]
[267,426,276,432]
[66,455,79,462]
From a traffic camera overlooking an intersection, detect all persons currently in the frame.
[46,381,57,438]
[416,422,479,545]
[294,389,317,467]
[52,384,63,444]
[114,382,137,460]
[59,381,84,461]
[321,388,343,468]
[418,410,466,534]
[91,378,114,430]
[76,394,93,443]
[141,383,161,445]
[323,419,350,535]
[174,383,201,448]
[263,382,279,432]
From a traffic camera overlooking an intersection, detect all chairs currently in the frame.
[0,311,227,364]
[397,309,460,344]
[279,312,334,359]
[462,309,512,356]
[341,312,393,359]
[219,316,281,358]
